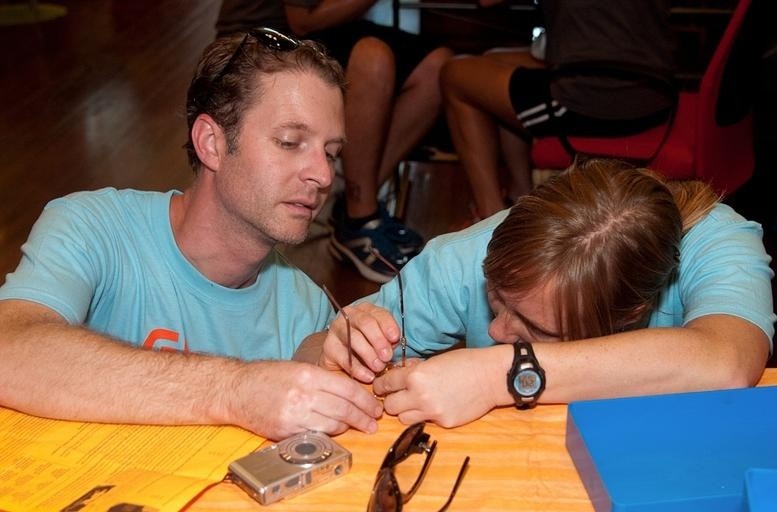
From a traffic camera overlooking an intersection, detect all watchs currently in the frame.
[506,340,546,410]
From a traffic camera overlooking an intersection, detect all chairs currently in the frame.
[531,0,753,200]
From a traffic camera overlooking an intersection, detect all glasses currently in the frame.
[322,247,408,401]
[202,26,298,112]
[366,421,471,512]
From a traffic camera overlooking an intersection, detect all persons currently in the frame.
[438,0,679,233]
[290,156,777,431]
[0,25,384,444]
[214,0,455,284]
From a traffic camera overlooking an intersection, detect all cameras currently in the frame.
[229,428,354,506]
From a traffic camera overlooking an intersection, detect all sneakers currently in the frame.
[325,190,426,285]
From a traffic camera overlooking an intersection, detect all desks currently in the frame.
[0,354,777,512]
